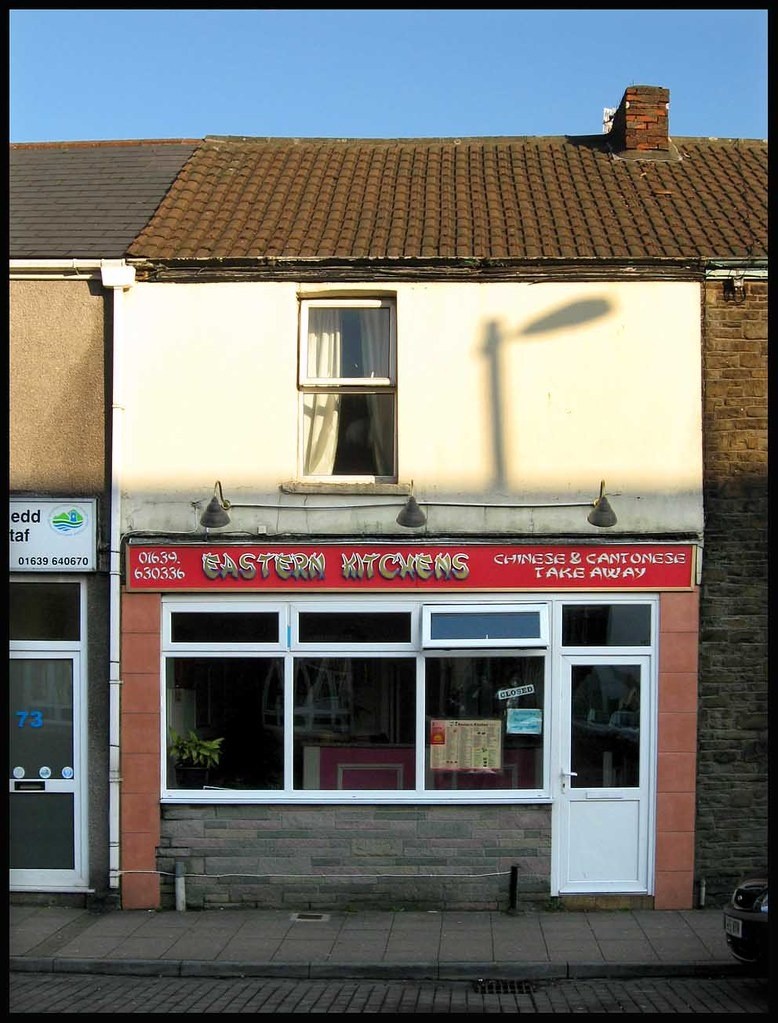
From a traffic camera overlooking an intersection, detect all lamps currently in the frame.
[588,481,618,527]
[395,478,428,528]
[198,482,233,529]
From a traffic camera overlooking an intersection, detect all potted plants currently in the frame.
[170,731,223,788]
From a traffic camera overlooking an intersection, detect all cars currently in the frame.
[723,865,768,967]
